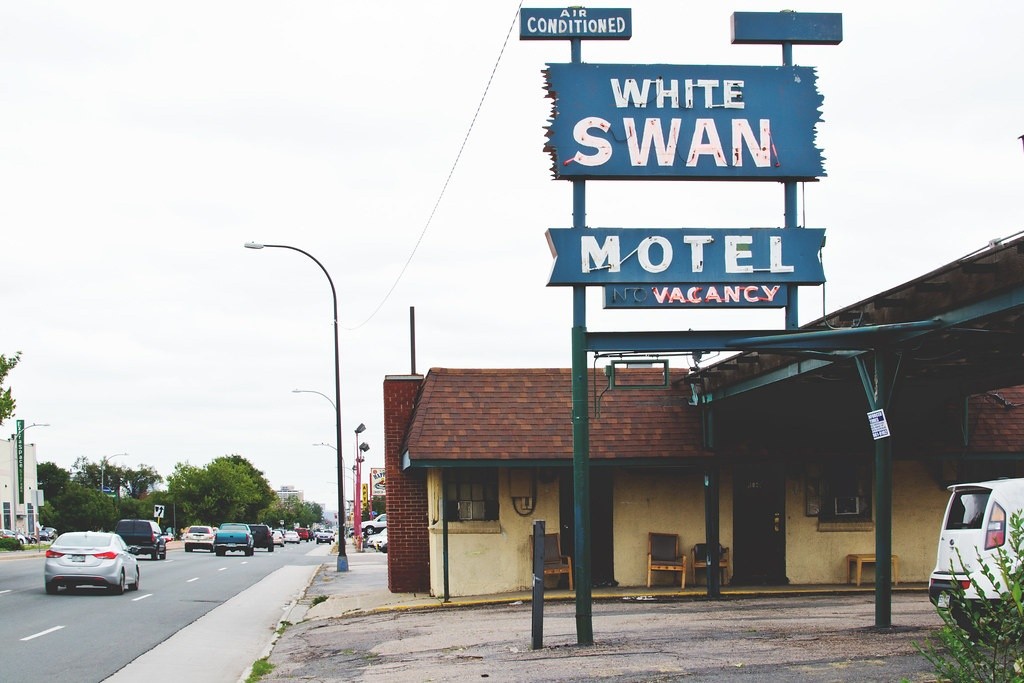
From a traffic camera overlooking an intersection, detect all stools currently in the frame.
[847,554,898,587]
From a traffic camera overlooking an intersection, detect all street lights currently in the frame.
[246,243,348,573]
[101,453,127,492]
[12,423,50,540]
[352,424,371,553]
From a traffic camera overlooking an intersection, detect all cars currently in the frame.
[271,530,284,547]
[367,527,388,554]
[284,532,300,544]
[361,514,387,535]
[116,519,167,561]
[249,525,274,552]
[39,531,49,542]
[294,528,335,545]
[46,529,58,539]
[184,526,215,552]
[928,478,1024,644]
[44,531,140,596]
[0,528,37,545]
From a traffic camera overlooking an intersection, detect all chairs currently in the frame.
[691,542,733,587]
[647,532,688,590]
[529,533,574,591]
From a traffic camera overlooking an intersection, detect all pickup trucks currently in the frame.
[215,523,256,556]
